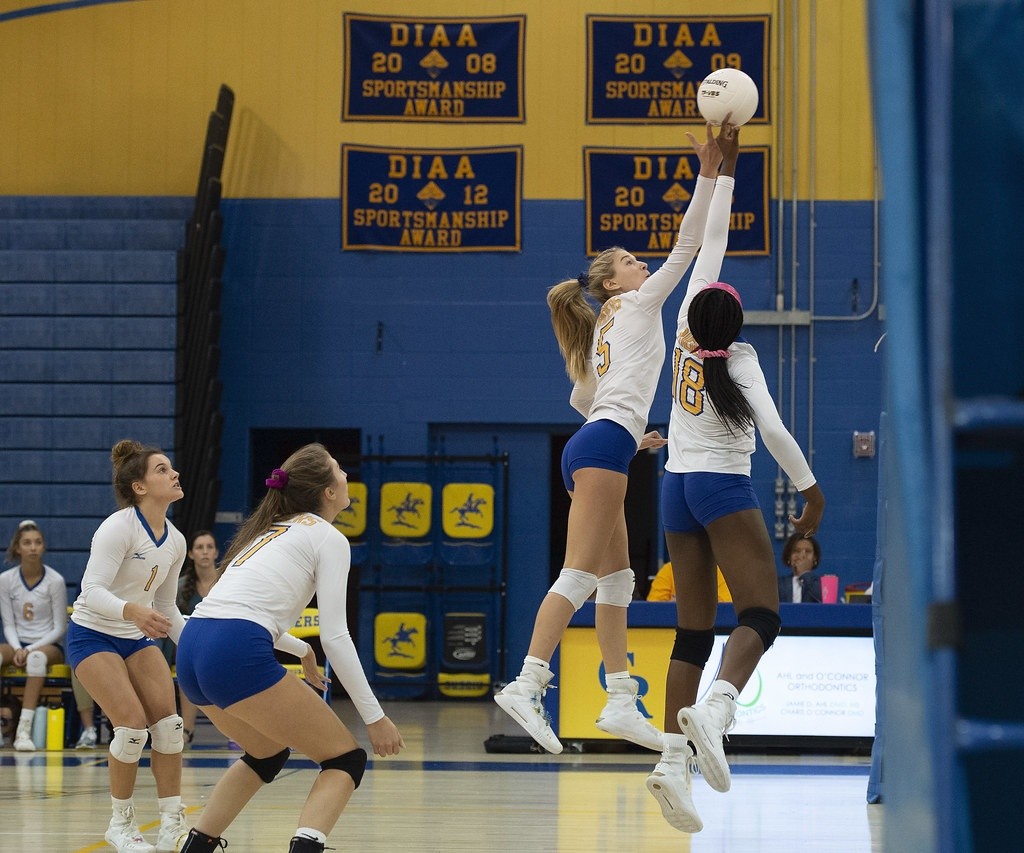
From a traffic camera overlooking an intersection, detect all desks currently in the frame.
[542,601,878,757]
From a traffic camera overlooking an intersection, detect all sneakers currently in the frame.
[156,810,190,852]
[493,658,562,754]
[76,726,97,749]
[105,809,156,853]
[644,743,702,833]
[597,682,669,751]
[678,696,736,793]
[13,731,36,751]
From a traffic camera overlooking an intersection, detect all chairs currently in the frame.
[0,607,75,749]
[279,607,331,706]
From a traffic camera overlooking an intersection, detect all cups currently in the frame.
[46,708,63,750]
[821,576,838,603]
[31,707,47,749]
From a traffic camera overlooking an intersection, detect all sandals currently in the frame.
[183,729,193,750]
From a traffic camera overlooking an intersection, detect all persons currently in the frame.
[0,520,68,751]
[176,530,221,615]
[645,111,826,834]
[646,561,733,602]
[67,439,191,853]
[71,667,97,749]
[494,122,723,755]
[778,533,822,603]
[177,444,406,853]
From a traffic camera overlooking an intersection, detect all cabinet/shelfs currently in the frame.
[322,434,510,702]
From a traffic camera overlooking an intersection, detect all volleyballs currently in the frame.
[696,68,760,129]
